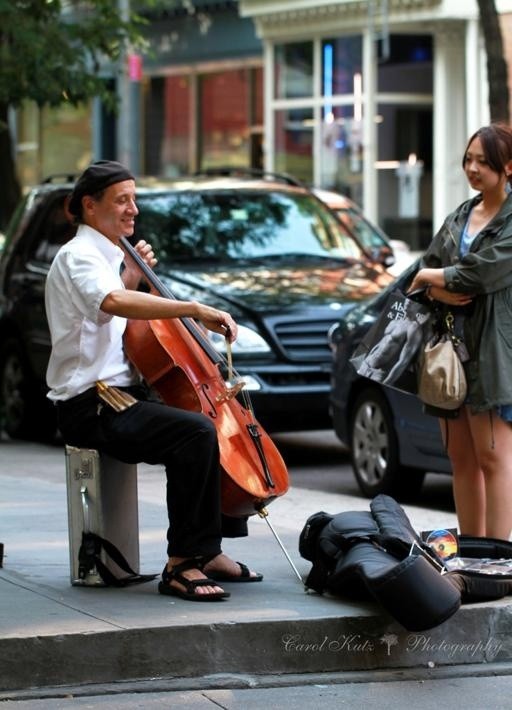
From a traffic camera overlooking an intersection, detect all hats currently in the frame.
[69,160,135,216]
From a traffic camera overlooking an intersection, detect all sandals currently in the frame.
[158,550,263,602]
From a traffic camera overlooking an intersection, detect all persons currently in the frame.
[43,158,263,600]
[403,120,512,544]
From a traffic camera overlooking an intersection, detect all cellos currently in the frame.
[118,235,290,517]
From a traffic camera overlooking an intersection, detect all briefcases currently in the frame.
[65,443,139,587]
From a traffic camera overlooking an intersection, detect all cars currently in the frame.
[328,250,452,500]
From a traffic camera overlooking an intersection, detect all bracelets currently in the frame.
[426,287,434,301]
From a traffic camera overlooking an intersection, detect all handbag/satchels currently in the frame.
[348,288,472,410]
